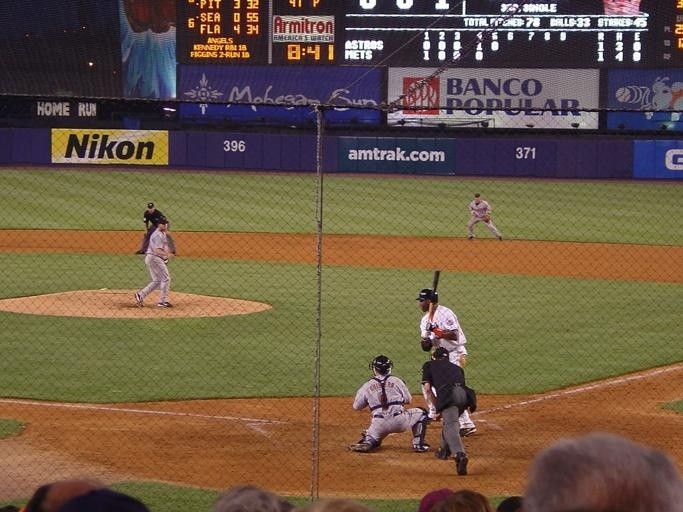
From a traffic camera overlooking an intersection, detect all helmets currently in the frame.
[431,347,450,361]
[369,355,394,376]
[415,289,438,302]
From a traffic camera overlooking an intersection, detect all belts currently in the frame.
[373,411,401,418]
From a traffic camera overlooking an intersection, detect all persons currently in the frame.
[466,192,502,241]
[415,288,478,438]
[345,355,432,453]
[1,480,148,512]
[202,430,683,511]
[135,215,173,308]
[419,346,477,475]
[136,203,176,257]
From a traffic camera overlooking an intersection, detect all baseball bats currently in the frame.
[428,271,439,322]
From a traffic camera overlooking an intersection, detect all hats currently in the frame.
[148,203,154,209]
[155,215,169,225]
[417,489,454,512]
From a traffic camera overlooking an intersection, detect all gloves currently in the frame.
[421,321,438,339]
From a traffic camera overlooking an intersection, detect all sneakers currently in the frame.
[414,444,430,452]
[429,413,442,421]
[135,250,144,255]
[158,302,172,307]
[460,427,477,437]
[349,442,370,453]
[435,447,447,460]
[134,293,144,307]
[456,454,468,475]
